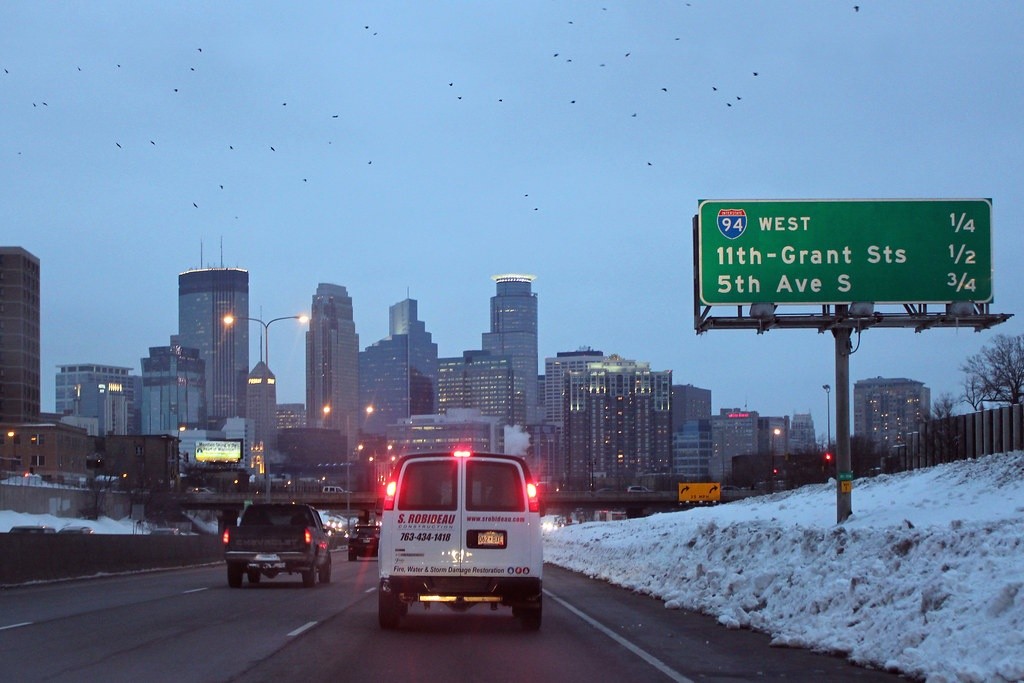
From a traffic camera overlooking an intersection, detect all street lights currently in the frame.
[591,461,596,489]
[822,385,831,483]
[770,427,781,492]
[222,314,311,496]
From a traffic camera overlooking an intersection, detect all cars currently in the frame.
[720,484,741,493]
[58,525,94,535]
[596,487,618,493]
[7,525,56,534]
[627,485,654,493]
[149,527,181,535]
[191,487,216,496]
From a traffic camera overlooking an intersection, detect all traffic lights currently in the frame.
[824,452,833,463]
[772,468,778,475]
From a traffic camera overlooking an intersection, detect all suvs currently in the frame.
[347,523,381,561]
[322,485,352,493]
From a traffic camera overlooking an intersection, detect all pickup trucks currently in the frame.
[222,501,336,588]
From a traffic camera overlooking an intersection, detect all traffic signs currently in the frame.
[678,482,720,502]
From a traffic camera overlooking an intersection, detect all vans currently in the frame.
[375,446,545,630]
